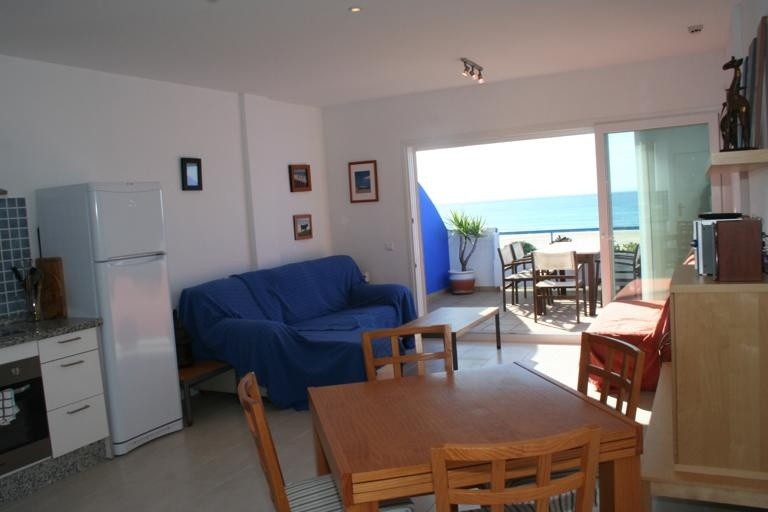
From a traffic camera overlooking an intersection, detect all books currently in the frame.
[691,216,764,283]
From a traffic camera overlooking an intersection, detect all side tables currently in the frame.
[179,360,239,427]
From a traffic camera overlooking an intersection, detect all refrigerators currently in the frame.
[32,179,185,458]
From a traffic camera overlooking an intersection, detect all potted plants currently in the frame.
[446,208,485,294]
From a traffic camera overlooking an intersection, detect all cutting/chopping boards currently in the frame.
[34,257,69,320]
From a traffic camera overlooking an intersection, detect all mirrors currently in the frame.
[180,157,203,191]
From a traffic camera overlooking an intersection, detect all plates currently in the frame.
[697,212,743,219]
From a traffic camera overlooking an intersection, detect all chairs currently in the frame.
[497,241,640,324]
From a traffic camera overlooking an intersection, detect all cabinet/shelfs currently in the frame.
[669,149,768,490]
[37,327,110,460]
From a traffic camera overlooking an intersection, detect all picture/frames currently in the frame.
[288,164,312,192]
[348,160,378,203]
[293,214,313,241]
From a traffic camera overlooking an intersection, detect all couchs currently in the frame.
[178,255,417,407]
[583,254,696,389]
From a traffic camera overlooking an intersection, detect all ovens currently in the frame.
[1,354,51,477]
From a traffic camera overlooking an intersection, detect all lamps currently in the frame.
[460,57,484,84]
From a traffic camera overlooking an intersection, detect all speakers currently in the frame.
[711,219,763,281]
